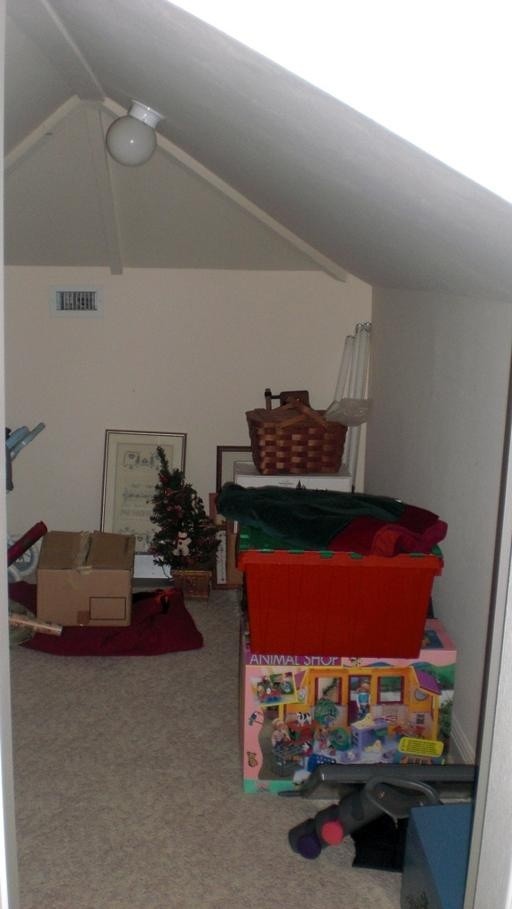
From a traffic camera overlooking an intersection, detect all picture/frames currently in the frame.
[99,429,188,556]
[215,446,256,495]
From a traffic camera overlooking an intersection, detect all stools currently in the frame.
[399,803,473,908]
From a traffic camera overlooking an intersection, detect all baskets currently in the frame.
[244,395,349,476]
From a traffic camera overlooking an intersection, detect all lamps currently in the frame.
[105,97,167,167]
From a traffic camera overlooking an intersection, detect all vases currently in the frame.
[171,569,212,603]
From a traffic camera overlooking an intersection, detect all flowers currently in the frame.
[145,443,222,581]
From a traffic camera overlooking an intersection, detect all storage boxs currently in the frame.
[242,616,457,796]
[232,461,353,536]
[36,531,136,628]
[235,533,441,658]
